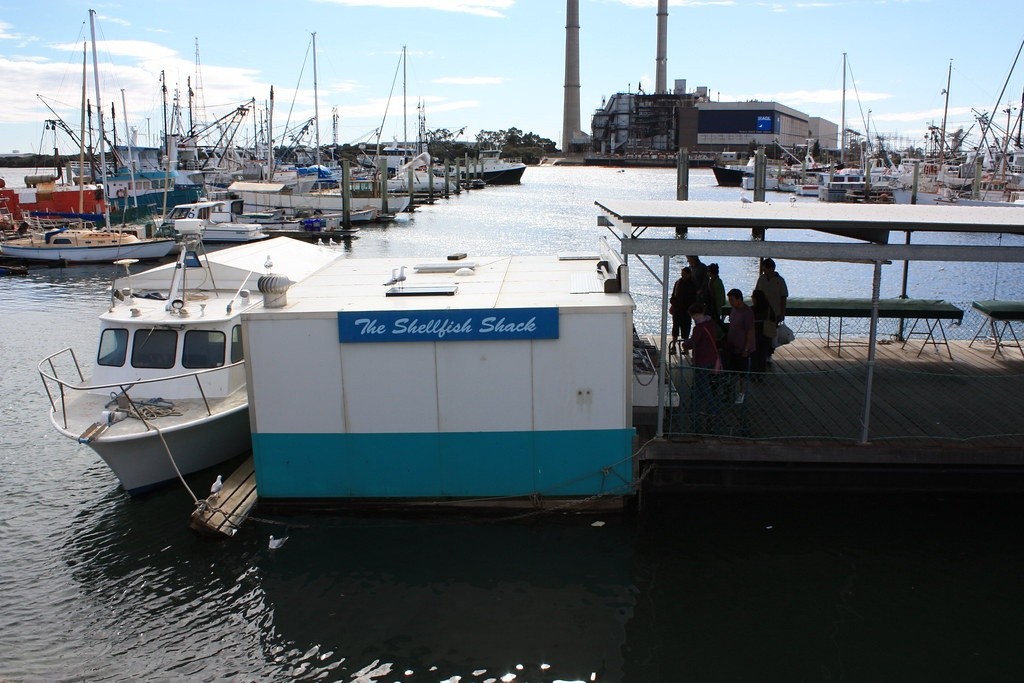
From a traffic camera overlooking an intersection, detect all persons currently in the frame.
[668,254,788,438]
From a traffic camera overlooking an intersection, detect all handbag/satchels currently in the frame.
[763,307,777,338]
[714,358,721,375]
[772,319,795,348]
[669,306,673,314]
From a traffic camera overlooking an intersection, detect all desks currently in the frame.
[720,298,964,361]
[968,300,1024,360]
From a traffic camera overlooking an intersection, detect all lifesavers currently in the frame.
[188,212,195,219]
[116,188,125,198]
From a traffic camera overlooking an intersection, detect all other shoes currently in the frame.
[669,347,676,355]
[681,349,689,354]
[735,393,745,403]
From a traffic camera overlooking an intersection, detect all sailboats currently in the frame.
[0,8,176,265]
[3,30,528,263]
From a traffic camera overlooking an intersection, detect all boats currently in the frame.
[711,38,1024,199]
[34,216,342,494]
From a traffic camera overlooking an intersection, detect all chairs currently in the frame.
[711,354,752,437]
